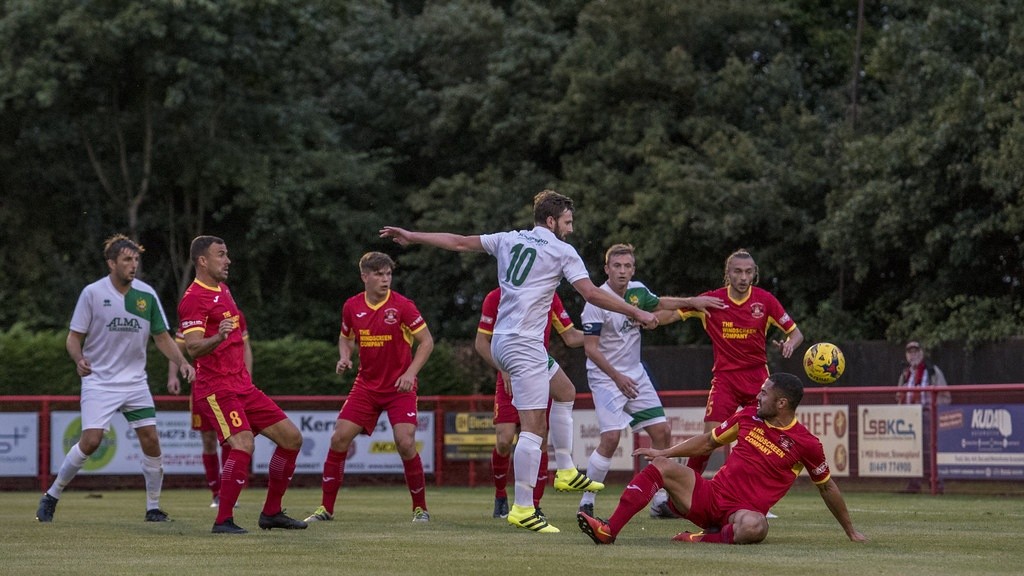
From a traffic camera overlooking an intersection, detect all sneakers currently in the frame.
[211,516,249,534]
[577,511,616,544]
[144,507,175,523]
[492,495,509,517]
[671,530,704,543]
[304,505,335,522]
[36,492,59,522]
[534,507,544,518]
[412,507,429,522]
[576,503,594,517]
[508,504,561,533]
[651,499,681,520]
[553,466,605,493]
[258,508,308,528]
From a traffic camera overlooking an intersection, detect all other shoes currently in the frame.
[209,494,219,506]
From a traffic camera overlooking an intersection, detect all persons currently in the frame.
[36,235,197,521]
[895,342,951,493]
[168,326,254,508]
[474,286,585,519]
[576,244,725,519]
[646,249,804,520]
[306,252,434,523]
[576,373,871,545]
[178,235,308,534]
[380,190,660,511]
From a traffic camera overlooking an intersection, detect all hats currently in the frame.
[905,341,920,349]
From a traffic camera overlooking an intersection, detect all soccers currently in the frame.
[803,342,846,385]
